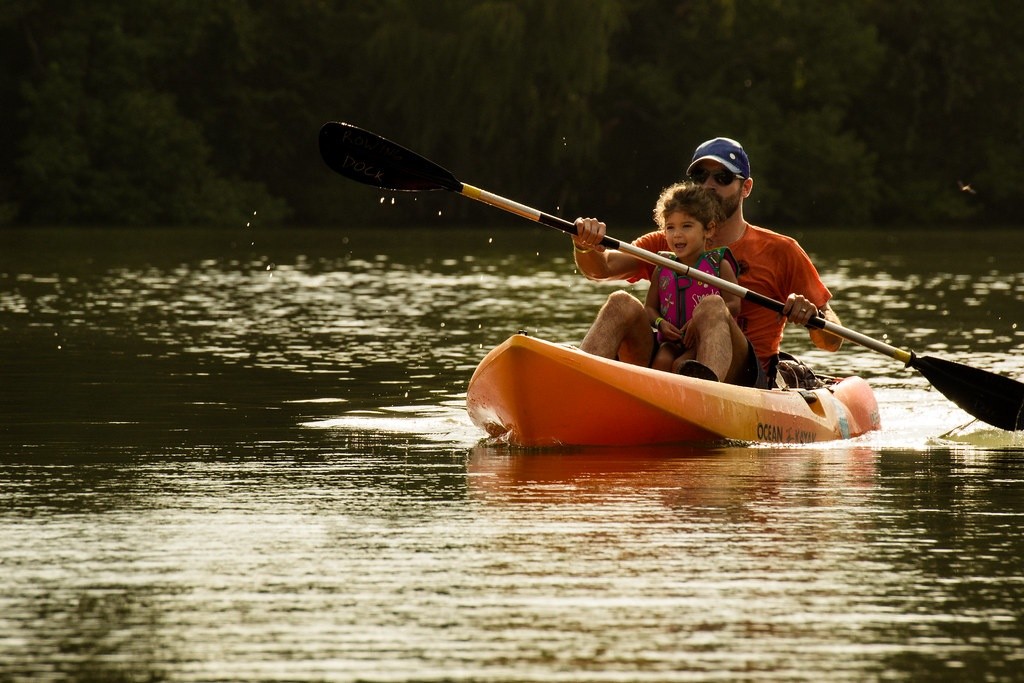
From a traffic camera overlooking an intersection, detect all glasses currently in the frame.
[689,167,746,185]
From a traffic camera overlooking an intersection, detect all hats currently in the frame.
[686,137,750,179]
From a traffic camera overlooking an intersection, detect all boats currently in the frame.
[462,330,894,451]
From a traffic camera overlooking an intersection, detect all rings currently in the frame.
[801,308,806,312]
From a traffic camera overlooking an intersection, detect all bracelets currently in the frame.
[654,317,663,327]
[573,239,593,254]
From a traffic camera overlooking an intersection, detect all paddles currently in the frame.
[317,120,1024,431]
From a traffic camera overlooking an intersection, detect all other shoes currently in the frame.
[675,360,719,382]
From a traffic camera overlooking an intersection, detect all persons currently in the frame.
[572,137,845,390]
[645,182,746,377]
